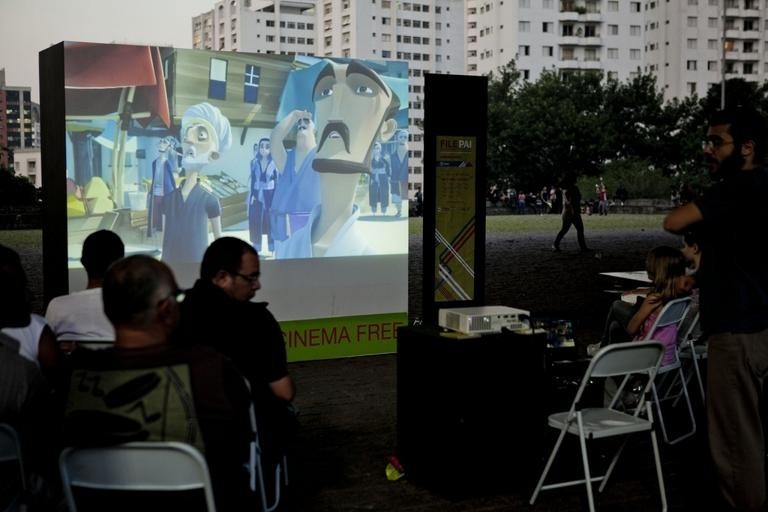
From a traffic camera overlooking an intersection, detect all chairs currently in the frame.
[664,311,709,409]
[530,340,668,512]
[0,422,27,510]
[58,441,216,512]
[242,372,289,512]
[633,297,697,445]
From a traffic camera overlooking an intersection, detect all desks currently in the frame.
[600,270,681,284]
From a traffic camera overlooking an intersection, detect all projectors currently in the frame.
[438,306,531,335]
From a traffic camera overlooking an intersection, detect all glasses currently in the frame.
[237,271,261,283]
[702,140,733,150]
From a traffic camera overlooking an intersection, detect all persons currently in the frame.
[268,108,324,242]
[551,178,593,253]
[157,101,232,263]
[248,137,282,260]
[595,176,608,215]
[414,186,423,217]
[388,130,409,217]
[1,230,312,511]
[663,104,767,512]
[273,57,403,259]
[368,140,391,216]
[146,135,178,237]
[588,245,697,391]
[680,230,702,280]
[488,182,562,215]
